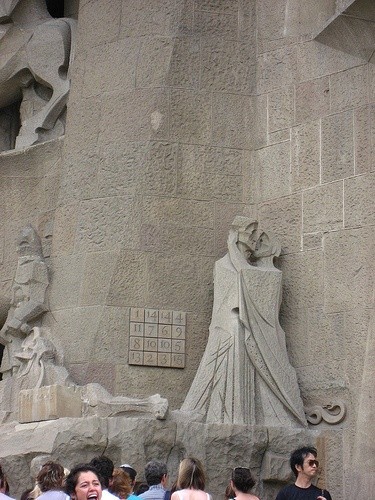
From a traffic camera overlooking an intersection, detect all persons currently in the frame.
[0,455,260,500]
[276,445,332,500]
[0,223,50,380]
[178,215,259,426]
[226,228,309,428]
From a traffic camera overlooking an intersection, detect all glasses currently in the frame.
[233,466,251,479]
[303,459,319,467]
